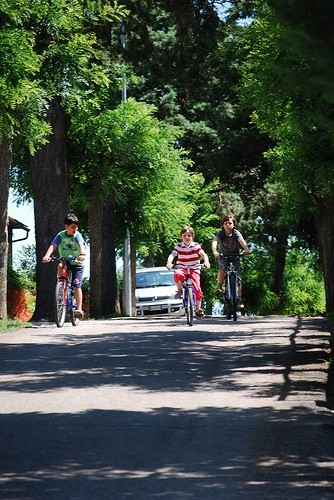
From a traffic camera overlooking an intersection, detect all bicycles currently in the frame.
[47,254,87,325]
[177,262,207,324]
[215,252,251,319]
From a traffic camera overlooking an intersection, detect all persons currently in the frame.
[41,213,86,319]
[166,226,210,320]
[211,215,250,309]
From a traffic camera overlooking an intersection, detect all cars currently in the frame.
[136,266,185,314]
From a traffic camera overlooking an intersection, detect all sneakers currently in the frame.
[216,288,223,296]
[197,309,202,320]
[74,309,84,318]
[237,300,244,309]
[175,291,183,299]
